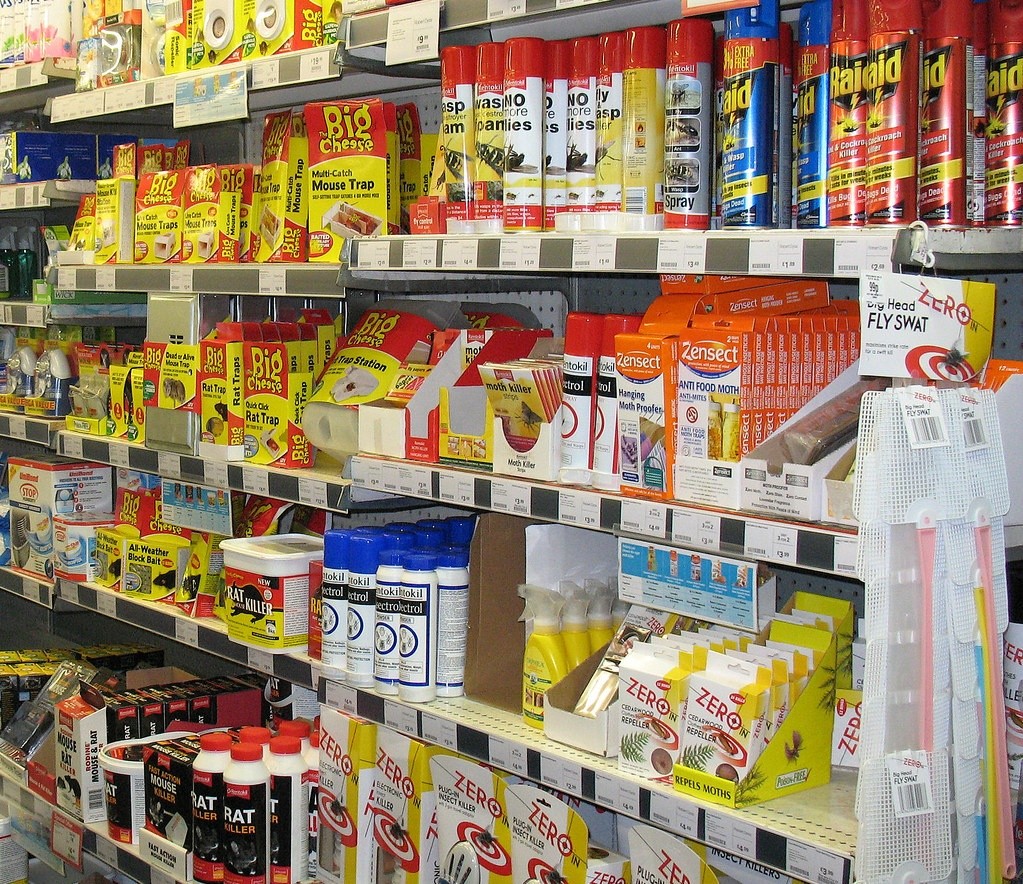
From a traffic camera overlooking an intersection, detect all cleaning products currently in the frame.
[515,575,632,732]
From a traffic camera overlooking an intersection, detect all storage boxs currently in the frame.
[172,682,213,725]
[93,682,139,743]
[43,646,74,663]
[171,738,202,755]
[191,678,228,725]
[140,684,187,731]
[125,641,165,668]
[164,676,262,734]
[115,690,165,739]
[0,663,17,734]
[17,650,48,664]
[141,741,200,853]
[213,678,254,693]
[104,643,136,672]
[39,662,61,688]
[54,678,108,824]
[76,644,114,668]
[9,664,46,711]
[8,454,112,584]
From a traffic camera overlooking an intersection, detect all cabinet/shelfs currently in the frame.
[0,0,1023,884]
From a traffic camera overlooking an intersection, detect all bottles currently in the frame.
[0,238,14,300]
[191,715,320,883]
[441,0,1023,231]
[14,240,37,299]
[320,513,474,704]
